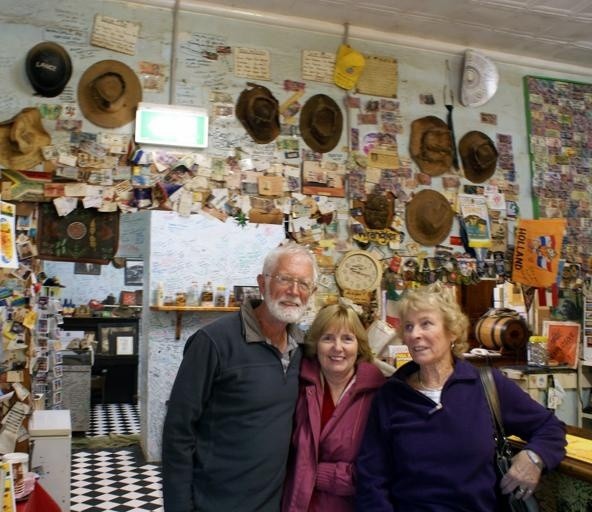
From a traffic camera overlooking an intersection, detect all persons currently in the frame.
[279,298,390,511]
[160,241,320,512]
[353,277,566,511]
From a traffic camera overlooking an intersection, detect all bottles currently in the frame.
[153,281,228,308]
[62,298,69,316]
[67,300,76,317]
[526,335,550,366]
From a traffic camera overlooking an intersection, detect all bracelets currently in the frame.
[523,447,543,471]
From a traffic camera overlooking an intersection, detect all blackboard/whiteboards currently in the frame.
[37,204,120,264]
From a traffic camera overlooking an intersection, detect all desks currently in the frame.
[456,347,592,427]
[506,424,592,512]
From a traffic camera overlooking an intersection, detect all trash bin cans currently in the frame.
[50,354,91,438]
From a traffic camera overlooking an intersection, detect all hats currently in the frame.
[77,60,143,130]
[236,82,281,143]
[300,94,342,152]
[409,116,498,183]
[333,44,365,89]
[405,189,453,245]
[0,42,73,173]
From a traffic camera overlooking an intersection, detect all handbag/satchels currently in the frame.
[494,435,540,512]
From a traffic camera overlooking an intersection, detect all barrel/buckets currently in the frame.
[474,311,528,350]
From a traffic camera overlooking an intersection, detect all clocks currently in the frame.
[335,250,383,291]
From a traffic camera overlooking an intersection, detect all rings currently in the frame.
[527,488,533,495]
[518,487,525,493]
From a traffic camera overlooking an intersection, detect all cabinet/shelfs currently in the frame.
[59,317,139,404]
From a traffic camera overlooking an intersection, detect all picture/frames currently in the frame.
[125,259,144,285]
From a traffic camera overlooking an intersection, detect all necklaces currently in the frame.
[416,367,454,392]
